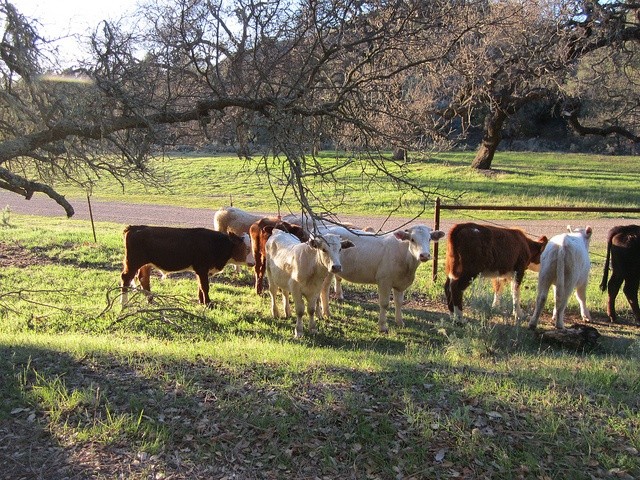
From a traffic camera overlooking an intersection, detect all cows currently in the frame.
[212,204,330,300]
[600,223,639,331]
[249,216,311,297]
[309,221,446,336]
[527,223,598,331]
[263,228,356,339]
[120,221,251,319]
[442,221,549,327]
